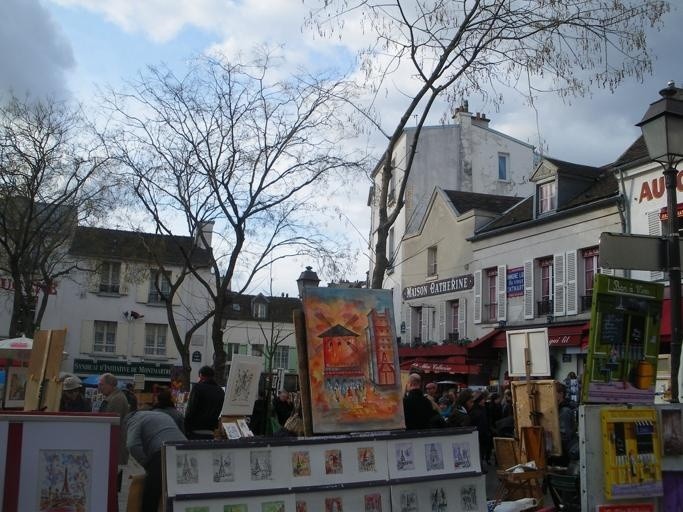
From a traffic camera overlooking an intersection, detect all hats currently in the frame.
[62,376,83,391]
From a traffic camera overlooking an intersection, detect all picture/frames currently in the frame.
[3,366,28,409]
[220,354,264,416]
[17,416,111,512]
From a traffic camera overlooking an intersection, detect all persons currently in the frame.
[123,407,188,511]
[546,380,579,467]
[402,371,515,467]
[57,365,305,473]
